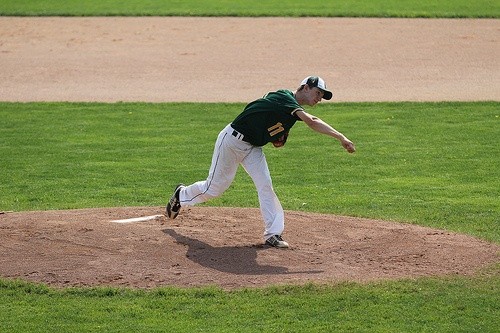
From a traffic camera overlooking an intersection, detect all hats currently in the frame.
[301,76,333,100]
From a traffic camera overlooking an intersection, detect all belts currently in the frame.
[232,130,246,141]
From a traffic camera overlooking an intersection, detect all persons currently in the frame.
[167,74,356,248]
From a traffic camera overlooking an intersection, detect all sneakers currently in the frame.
[166,182,187,220]
[265,235,289,248]
